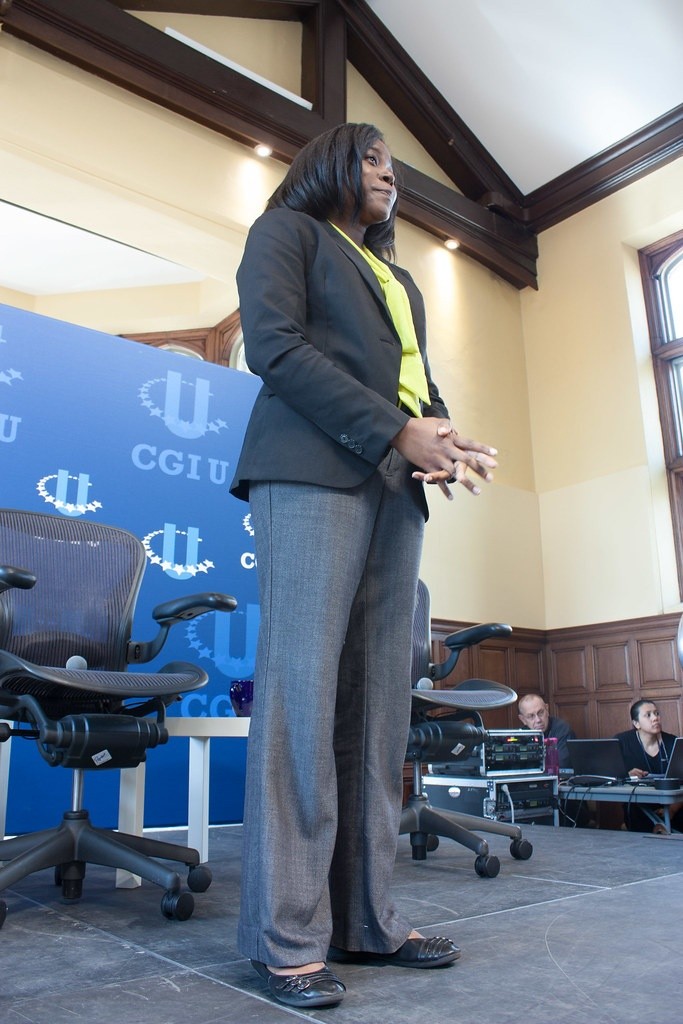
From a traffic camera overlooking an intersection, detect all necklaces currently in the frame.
[638,731,663,774]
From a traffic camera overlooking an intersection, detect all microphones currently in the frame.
[66,656,88,671]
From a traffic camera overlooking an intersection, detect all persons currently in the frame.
[229,123,497,1007]
[512,694,590,827]
[613,699,683,833]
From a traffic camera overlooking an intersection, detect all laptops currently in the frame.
[567,738,631,784]
[626,737,683,787]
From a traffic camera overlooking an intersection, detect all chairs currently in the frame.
[398,576,533,878]
[0,508,239,931]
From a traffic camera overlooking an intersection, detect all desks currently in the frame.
[112,715,250,889]
[558,784,681,834]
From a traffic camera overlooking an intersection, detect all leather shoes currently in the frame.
[328,936,463,966]
[249,958,347,1002]
